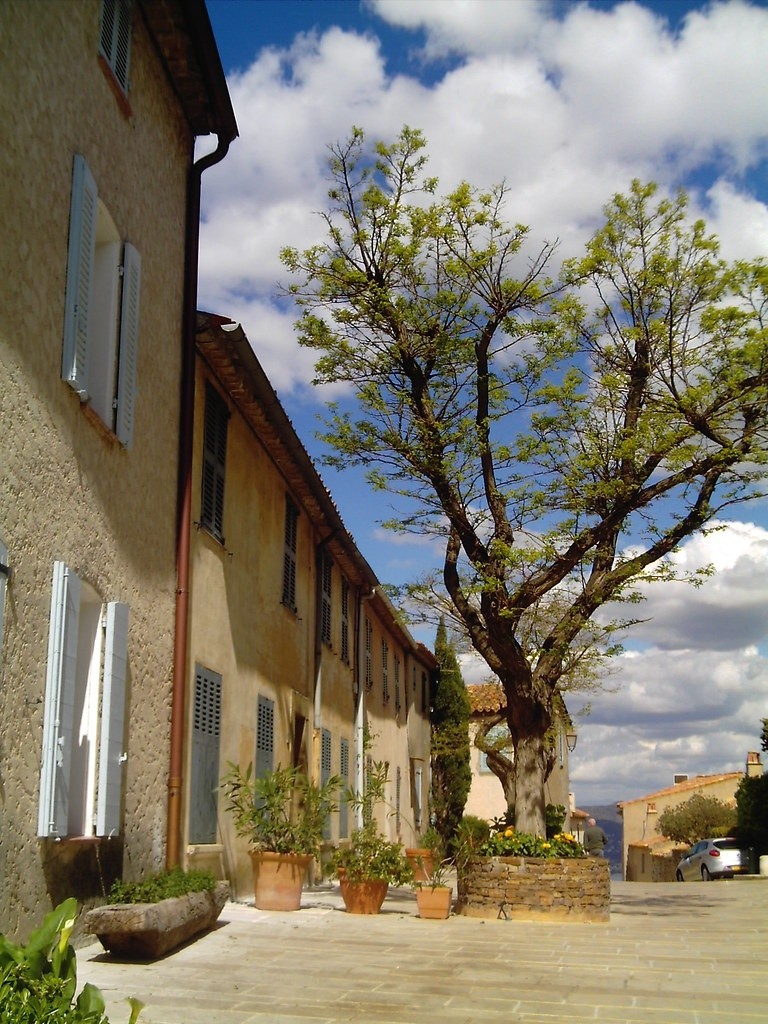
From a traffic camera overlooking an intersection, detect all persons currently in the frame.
[582,818,608,858]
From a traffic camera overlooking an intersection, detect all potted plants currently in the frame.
[211,760,475,919]
[84,868,231,961]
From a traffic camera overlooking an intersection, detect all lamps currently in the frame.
[566,735,577,752]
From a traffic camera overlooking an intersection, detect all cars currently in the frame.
[675,836,752,883]
